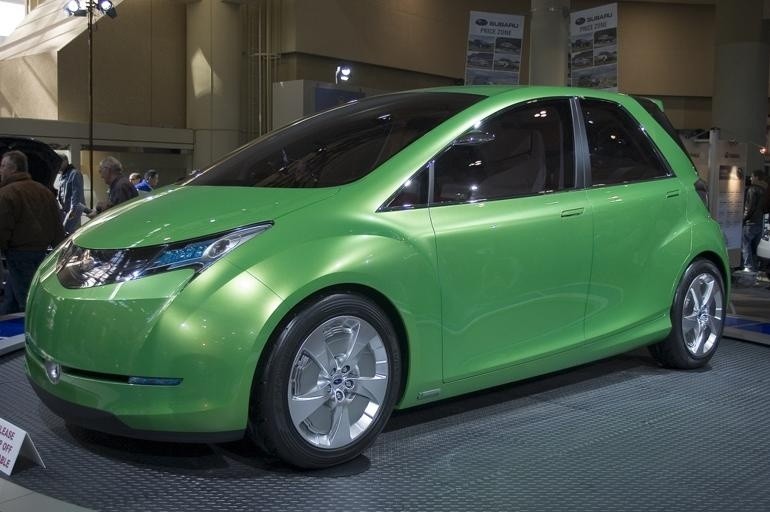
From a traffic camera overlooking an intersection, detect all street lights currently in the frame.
[65,0,117,209]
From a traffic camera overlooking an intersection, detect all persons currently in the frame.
[128,171,141,187]
[85,156,140,220]
[134,169,159,196]
[1,149,63,311]
[742,170,769,274]
[53,153,87,240]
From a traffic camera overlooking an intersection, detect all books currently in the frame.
[77,202,92,214]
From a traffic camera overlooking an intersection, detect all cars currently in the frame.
[756,213,770,276]
[18,77,735,471]
[496,58,512,66]
[573,31,614,68]
[472,38,491,49]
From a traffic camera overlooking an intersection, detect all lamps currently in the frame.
[62,0,119,21]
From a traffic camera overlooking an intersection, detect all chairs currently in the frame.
[460,127,546,200]
